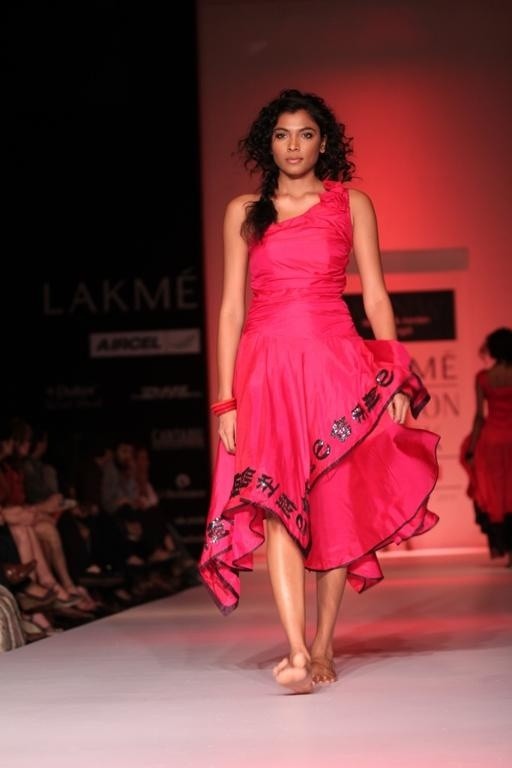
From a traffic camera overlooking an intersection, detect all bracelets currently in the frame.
[211,398,237,417]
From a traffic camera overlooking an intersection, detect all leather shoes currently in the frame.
[1,559,38,589]
[133,550,183,571]
[17,588,83,611]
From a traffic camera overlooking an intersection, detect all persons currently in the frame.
[198,89,441,693]
[0,417,197,651]
[459,327,512,567]
